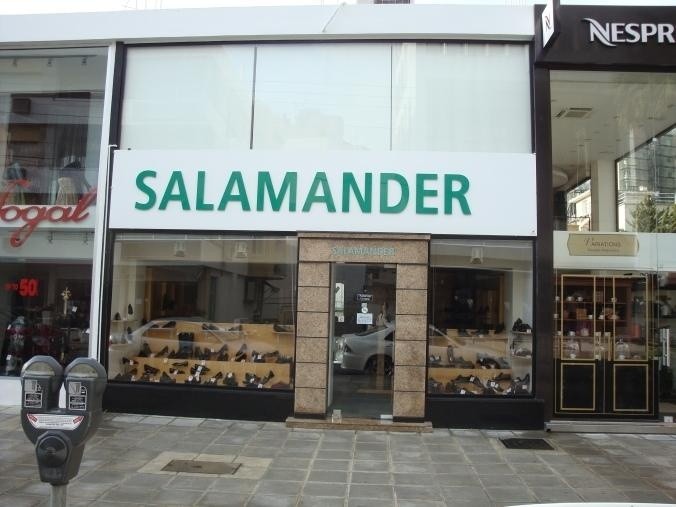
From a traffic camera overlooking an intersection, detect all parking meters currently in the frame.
[20,355,107,507]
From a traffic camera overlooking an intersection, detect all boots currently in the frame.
[173,332,194,359]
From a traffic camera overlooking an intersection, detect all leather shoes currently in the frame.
[110,334,126,344]
[224,370,289,389]
[512,319,532,332]
[274,325,292,332]
[114,357,223,387]
[115,312,121,320]
[126,326,133,340]
[142,318,158,328]
[511,347,532,356]
[202,323,217,330]
[139,343,151,357]
[428,344,510,369]
[194,343,292,363]
[427,371,529,395]
[128,304,133,314]
[228,324,241,331]
[155,346,174,358]
[161,320,175,327]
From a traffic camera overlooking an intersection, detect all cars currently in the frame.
[333,319,507,376]
[126,317,284,359]
[552,334,646,361]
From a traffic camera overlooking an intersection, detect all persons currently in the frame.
[376,303,391,326]
[58,156,91,204]
[8,163,27,206]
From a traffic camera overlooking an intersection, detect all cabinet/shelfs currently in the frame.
[124,320,294,387]
[430,337,533,395]
[554,270,658,421]
